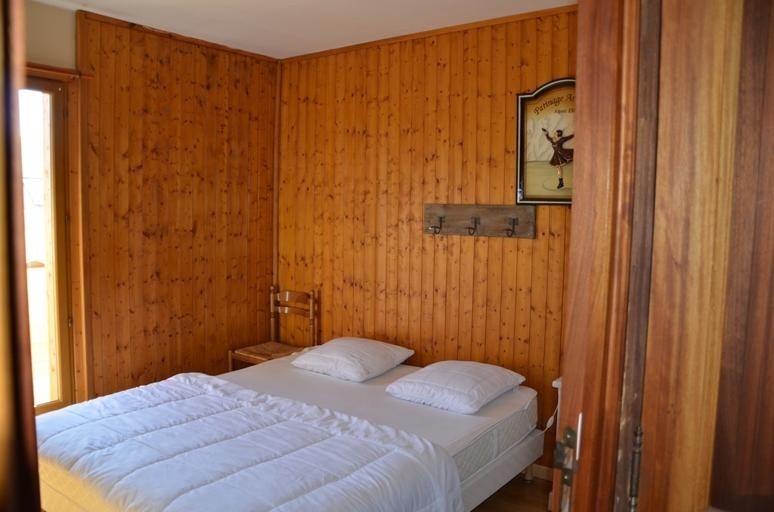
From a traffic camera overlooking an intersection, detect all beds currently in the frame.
[35,345,545,512]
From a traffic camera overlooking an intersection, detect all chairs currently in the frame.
[228,284,315,372]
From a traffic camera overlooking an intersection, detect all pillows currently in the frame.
[291,337,415,383]
[385,360,526,414]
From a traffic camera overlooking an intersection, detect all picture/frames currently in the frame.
[517,78,576,206]
